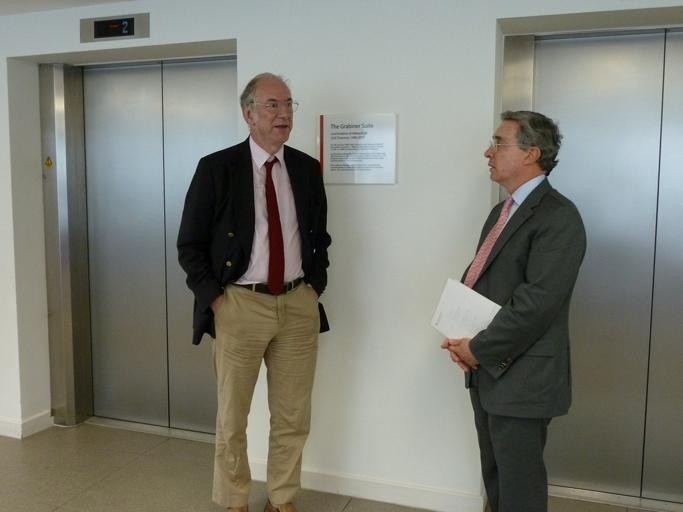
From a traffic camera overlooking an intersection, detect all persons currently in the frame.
[440,109,588,512]
[173,71,330,510]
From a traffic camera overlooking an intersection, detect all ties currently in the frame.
[464,197,513,287]
[265,159,285,294]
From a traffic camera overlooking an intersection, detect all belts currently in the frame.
[245,279,302,294]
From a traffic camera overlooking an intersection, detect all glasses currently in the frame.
[254,102,299,113]
[491,141,521,151]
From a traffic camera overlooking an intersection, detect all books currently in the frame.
[430,278,503,349]
